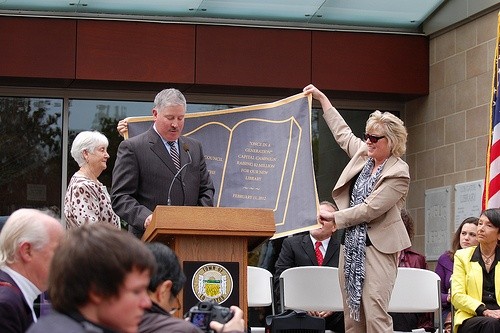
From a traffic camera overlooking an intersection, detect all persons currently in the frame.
[0,209,245,333]
[64,131,121,232]
[435,209,500,333]
[111,89,215,238]
[274,201,345,333]
[304,84,427,333]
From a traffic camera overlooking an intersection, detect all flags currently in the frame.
[481,41,500,212]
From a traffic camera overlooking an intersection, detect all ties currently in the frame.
[167,140,182,179]
[313,241,324,266]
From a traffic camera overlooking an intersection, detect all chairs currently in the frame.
[247,266,275,315]
[387,267,443,333]
[279,267,345,314]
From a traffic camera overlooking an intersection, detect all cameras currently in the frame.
[190,301,234,333]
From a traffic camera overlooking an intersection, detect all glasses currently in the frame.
[168,288,181,315]
[363,133,386,143]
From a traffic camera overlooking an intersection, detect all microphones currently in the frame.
[167,144,192,206]
